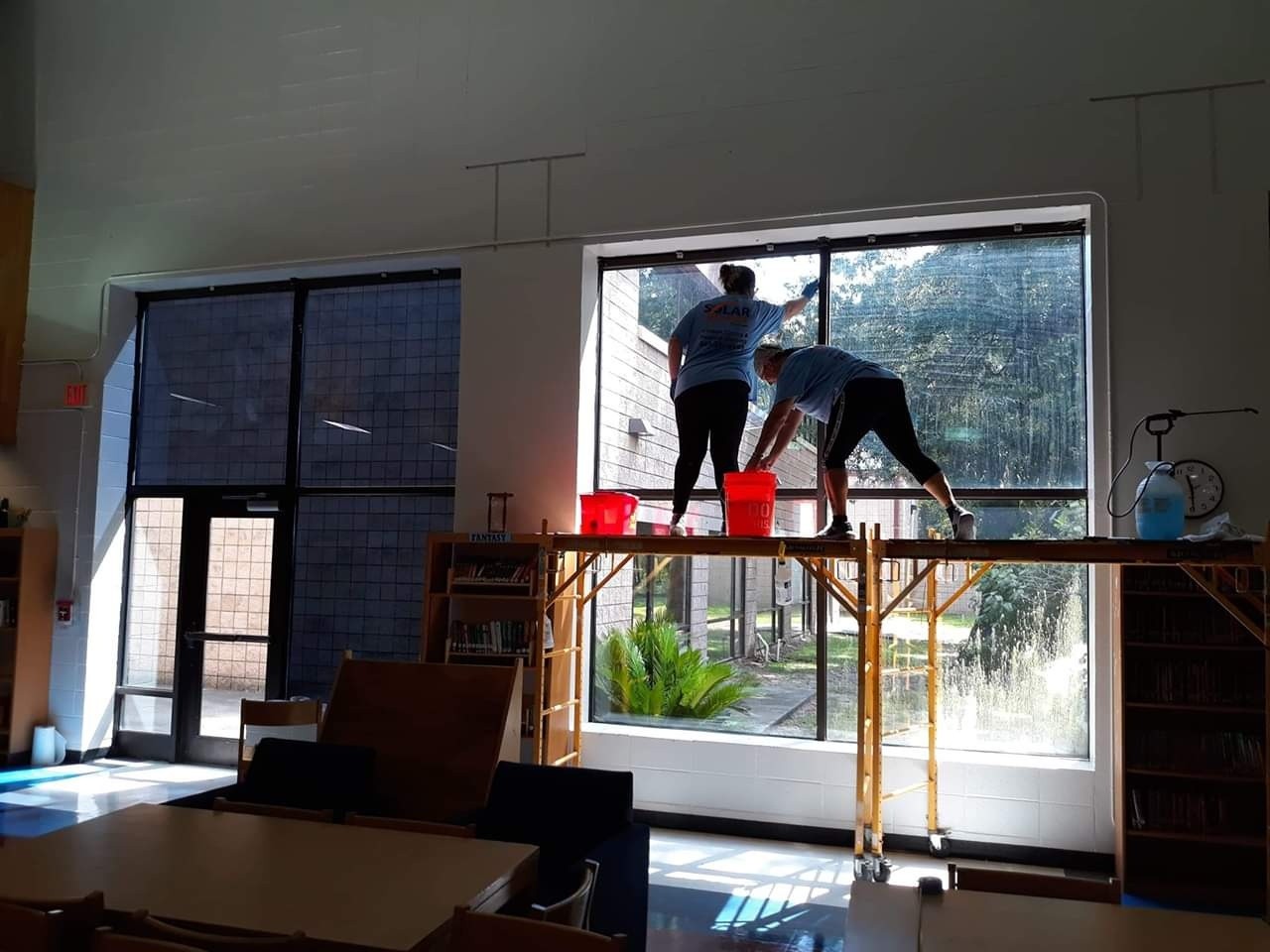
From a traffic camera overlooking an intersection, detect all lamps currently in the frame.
[628,418,656,437]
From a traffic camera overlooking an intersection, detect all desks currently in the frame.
[843,879,1270,952]
[0,803,541,952]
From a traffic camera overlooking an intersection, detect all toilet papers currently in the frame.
[31,724,68,766]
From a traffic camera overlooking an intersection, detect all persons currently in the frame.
[744,344,976,541]
[668,263,820,536]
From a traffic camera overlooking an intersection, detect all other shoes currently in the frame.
[670,514,687,537]
[813,521,855,539]
[952,506,975,539]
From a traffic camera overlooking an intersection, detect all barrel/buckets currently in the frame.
[579,491,638,535]
[722,471,778,537]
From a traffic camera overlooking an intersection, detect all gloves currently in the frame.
[802,278,819,299]
[670,379,677,402]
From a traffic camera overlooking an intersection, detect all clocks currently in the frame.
[1174,459,1225,519]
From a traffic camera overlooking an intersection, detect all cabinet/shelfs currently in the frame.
[418,531,586,767]
[0,525,59,768]
[1112,563,1270,913]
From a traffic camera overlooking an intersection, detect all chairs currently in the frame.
[237,699,323,784]
[125,907,306,952]
[446,903,628,952]
[162,737,390,816]
[528,857,601,931]
[947,863,1122,907]
[477,759,649,952]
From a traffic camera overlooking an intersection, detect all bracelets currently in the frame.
[752,452,763,458]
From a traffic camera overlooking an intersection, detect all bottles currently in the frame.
[1134,461,1186,540]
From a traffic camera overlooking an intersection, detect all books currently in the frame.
[451,619,536,653]
[455,559,530,584]
[1128,730,1264,834]
[1125,569,1263,648]
[1129,657,1264,710]
[521,707,533,737]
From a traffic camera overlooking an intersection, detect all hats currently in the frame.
[754,344,782,378]
